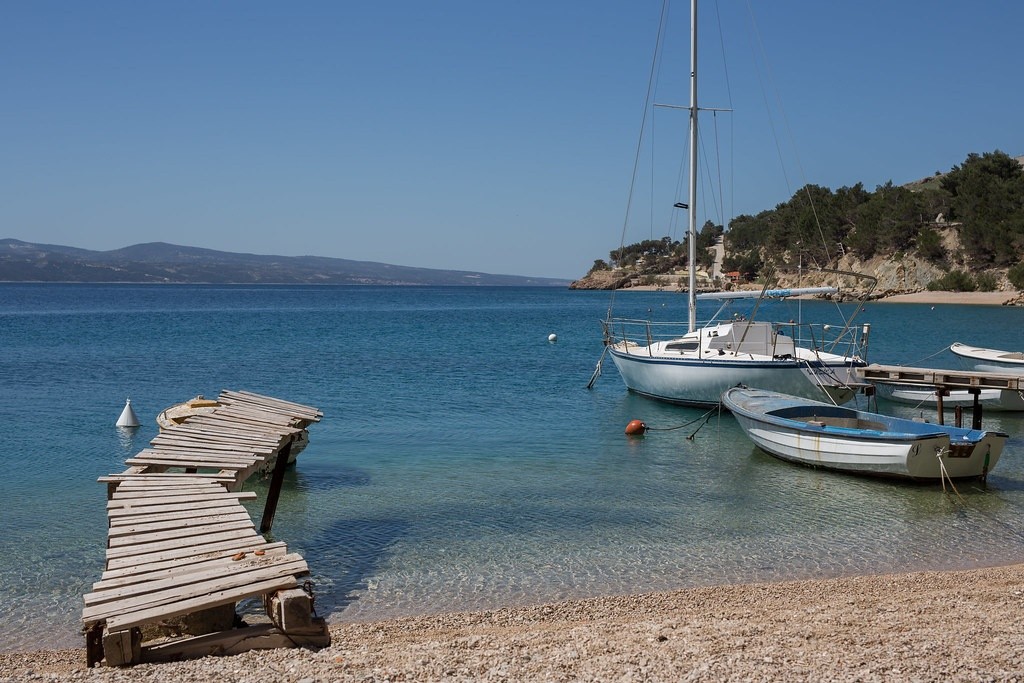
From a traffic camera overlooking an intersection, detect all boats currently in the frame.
[720,382,1010,480]
[868,381,1024,411]
[949,341,1024,376]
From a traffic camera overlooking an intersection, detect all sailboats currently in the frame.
[599,1,877,408]
